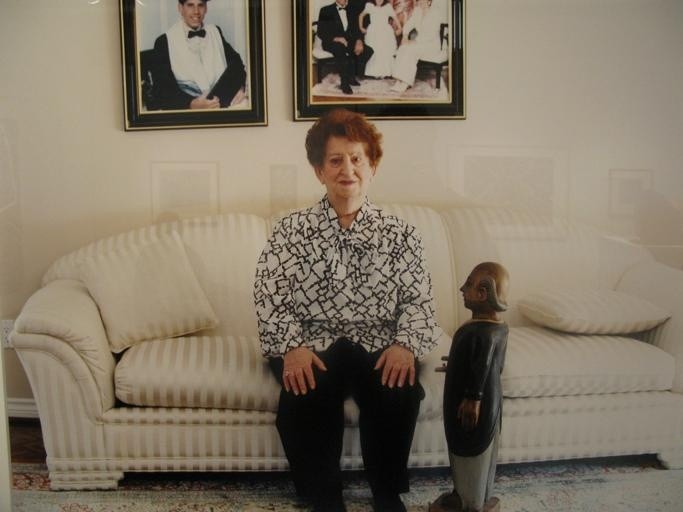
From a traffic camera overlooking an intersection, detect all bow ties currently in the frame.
[186,29,206,39]
[338,6,346,10]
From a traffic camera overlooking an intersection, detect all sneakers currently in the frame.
[339,76,360,95]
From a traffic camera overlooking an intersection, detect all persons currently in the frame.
[357,0,400,79]
[154,0,248,113]
[435,259,512,511]
[252,111,435,510]
[387,0,449,95]
[316,1,375,94]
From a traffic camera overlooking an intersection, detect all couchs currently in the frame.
[7,203,681,490]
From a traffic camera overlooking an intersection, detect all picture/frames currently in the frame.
[290,1,467,122]
[116,0,270,132]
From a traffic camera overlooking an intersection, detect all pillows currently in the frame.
[518,286,672,338]
[85,228,221,355]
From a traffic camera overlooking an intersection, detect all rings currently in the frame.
[283,370,292,376]
